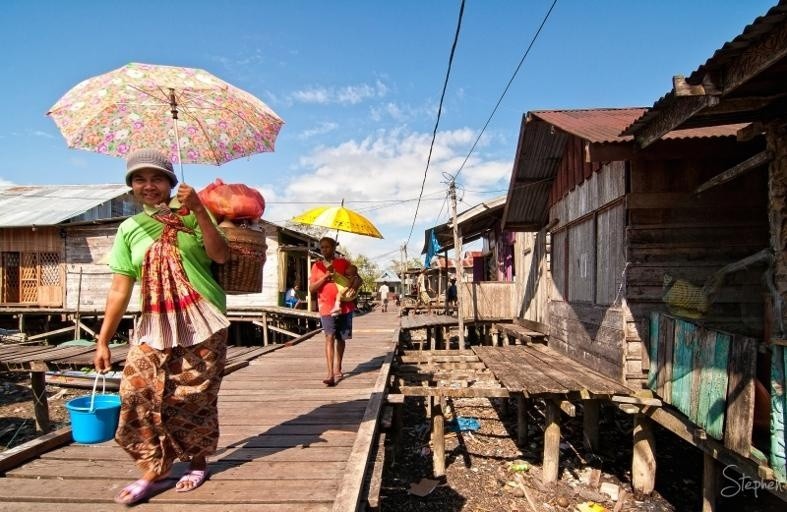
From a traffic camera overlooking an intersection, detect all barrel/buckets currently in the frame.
[67,370,120,442]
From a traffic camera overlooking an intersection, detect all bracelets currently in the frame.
[319,277,326,282]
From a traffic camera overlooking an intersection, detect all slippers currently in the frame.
[175,465,209,492]
[322,374,344,386]
[113,478,173,504]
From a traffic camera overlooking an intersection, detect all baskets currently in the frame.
[211,226,268,296]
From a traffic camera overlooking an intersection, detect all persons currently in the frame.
[94,148,230,505]
[283,279,303,310]
[330,265,358,317]
[412,266,432,318]
[447,278,457,305]
[378,281,390,313]
[308,237,362,385]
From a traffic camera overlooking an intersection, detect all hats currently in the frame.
[125,149,178,188]
[421,267,426,272]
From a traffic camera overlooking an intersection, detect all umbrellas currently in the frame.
[289,198,384,263]
[45,61,286,185]
